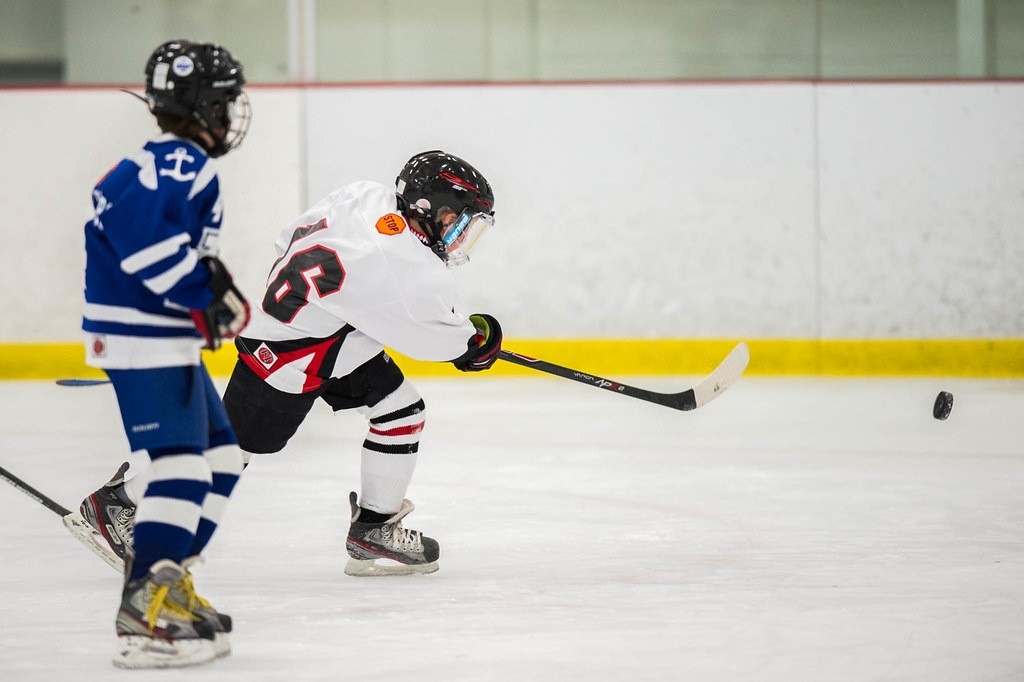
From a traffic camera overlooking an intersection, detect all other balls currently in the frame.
[933,390,953,422]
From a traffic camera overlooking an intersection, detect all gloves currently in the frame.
[191,256,250,351]
[452,314,503,371]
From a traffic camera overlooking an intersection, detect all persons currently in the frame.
[81,39,252,671]
[62,150,502,577]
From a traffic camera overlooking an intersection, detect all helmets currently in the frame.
[394,149,495,268]
[145,39,252,158]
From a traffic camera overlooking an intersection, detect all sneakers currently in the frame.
[170,555,232,657]
[344,491,440,576]
[61,462,138,575]
[112,559,216,669]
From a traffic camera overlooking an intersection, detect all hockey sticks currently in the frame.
[496,336,754,412]
[0,460,233,635]
